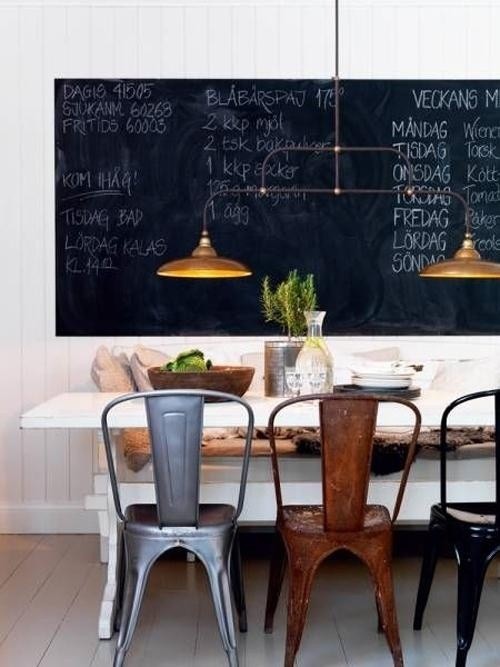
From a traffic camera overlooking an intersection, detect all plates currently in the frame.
[350,370,415,389]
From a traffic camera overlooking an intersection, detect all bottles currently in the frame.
[293,309,335,405]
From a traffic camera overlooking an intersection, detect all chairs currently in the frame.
[414,389,500,667]
[264,395,421,667]
[101,389,255,667]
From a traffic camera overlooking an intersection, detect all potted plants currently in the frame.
[261,270,322,398]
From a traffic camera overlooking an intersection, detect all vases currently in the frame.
[295,310,334,395]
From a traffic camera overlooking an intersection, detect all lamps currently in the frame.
[157,0,499,278]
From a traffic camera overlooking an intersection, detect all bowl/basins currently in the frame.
[147,364,255,403]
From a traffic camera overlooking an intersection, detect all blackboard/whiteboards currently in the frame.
[54,79,500,336]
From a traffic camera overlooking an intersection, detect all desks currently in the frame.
[19,393,497,639]
